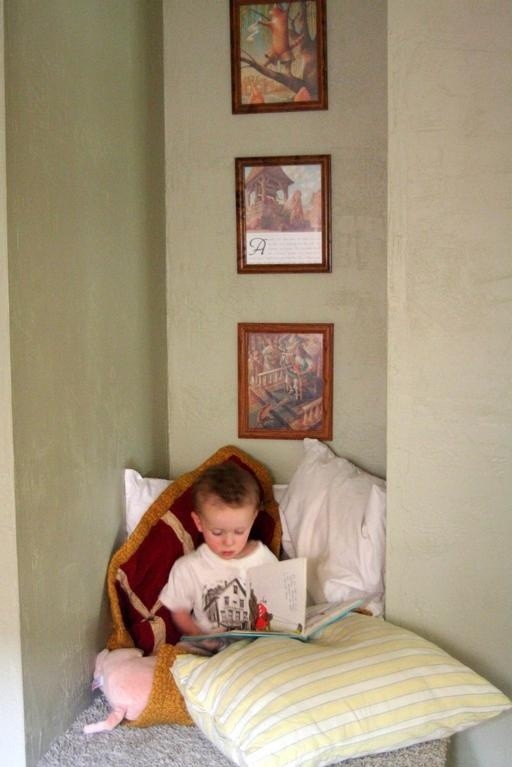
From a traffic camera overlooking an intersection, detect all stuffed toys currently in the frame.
[81,642,210,736]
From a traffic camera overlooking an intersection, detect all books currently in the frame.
[178,556,369,644]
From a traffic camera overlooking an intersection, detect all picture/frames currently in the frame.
[229,5,327,113]
[237,322,335,443]
[234,152,333,274]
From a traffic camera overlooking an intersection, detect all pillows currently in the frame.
[274,437,384,619]
[123,468,305,660]
[167,611,512,766]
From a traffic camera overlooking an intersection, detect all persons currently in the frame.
[155,458,285,661]
